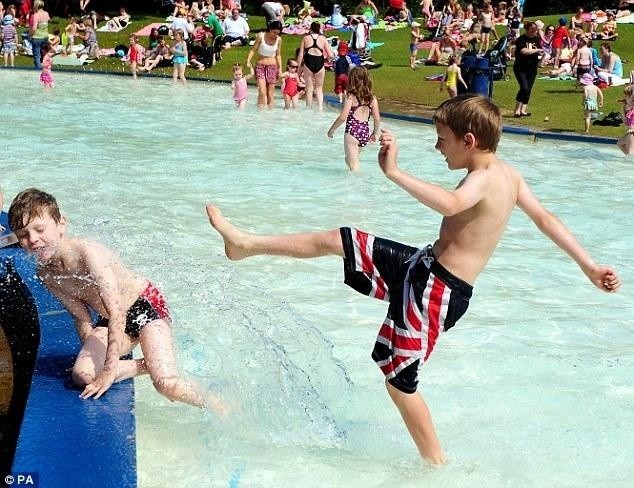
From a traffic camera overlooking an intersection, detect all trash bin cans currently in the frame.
[457,56,496,96]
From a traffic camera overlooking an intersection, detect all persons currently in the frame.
[208,89,622,464]
[7,187,230,422]
[0,1,633,156]
[327,65,382,174]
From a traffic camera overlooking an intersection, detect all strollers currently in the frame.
[461,34,511,84]
[419,0,447,41]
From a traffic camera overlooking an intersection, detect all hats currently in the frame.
[579,72,594,85]
[2,14,15,24]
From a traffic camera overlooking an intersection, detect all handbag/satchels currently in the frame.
[603,111,624,124]
